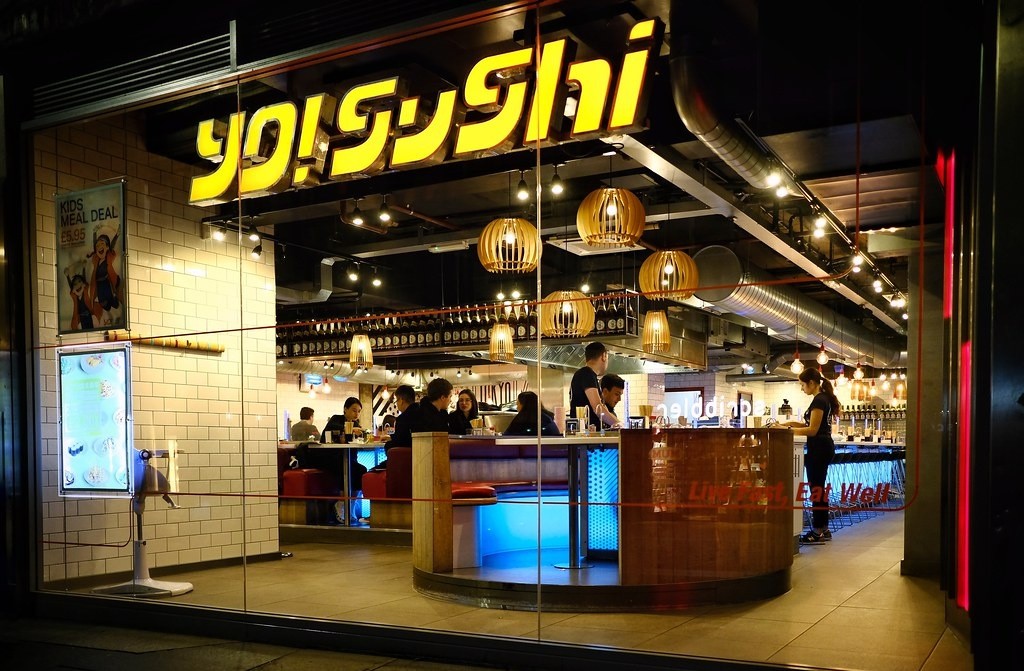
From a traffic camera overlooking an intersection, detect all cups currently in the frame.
[644,416,649,429]
[577,418,586,432]
[325,430,394,444]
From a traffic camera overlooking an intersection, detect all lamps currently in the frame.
[816,307,829,365]
[213,220,262,258]
[576,153,645,248]
[550,165,562,194]
[477,171,543,274]
[642,301,670,355]
[309,385,315,399]
[349,332,373,369]
[790,301,805,375]
[638,202,699,300]
[542,291,595,338]
[349,263,382,287]
[353,199,364,225]
[321,374,331,394]
[489,323,514,361]
[378,195,391,221]
[516,170,529,200]
[838,318,878,396]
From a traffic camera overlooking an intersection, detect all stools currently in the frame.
[804,451,905,535]
[90,445,193,596]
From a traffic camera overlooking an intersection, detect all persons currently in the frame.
[772,368,841,545]
[291,407,320,441]
[359,378,479,523]
[568,340,626,432]
[320,397,367,523]
[503,391,561,435]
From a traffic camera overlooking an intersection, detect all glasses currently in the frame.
[393,399,401,406]
[459,398,472,403]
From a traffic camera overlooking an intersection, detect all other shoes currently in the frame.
[358,518,370,524]
[335,501,344,520]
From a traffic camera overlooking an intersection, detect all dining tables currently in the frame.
[309,443,381,527]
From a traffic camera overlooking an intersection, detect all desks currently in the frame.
[496,436,619,570]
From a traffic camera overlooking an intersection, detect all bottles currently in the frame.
[649,416,656,429]
[276,290,634,359]
[839,404,907,420]
[483,425,495,436]
[590,424,596,432]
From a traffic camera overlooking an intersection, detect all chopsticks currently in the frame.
[344,422,354,434]
[576,407,586,418]
[469,419,483,428]
[638,405,653,417]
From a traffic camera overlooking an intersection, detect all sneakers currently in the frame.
[824,528,833,541]
[798,530,826,545]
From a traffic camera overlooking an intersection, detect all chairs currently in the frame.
[449,437,567,567]
[277,444,323,525]
[362,448,412,529]
